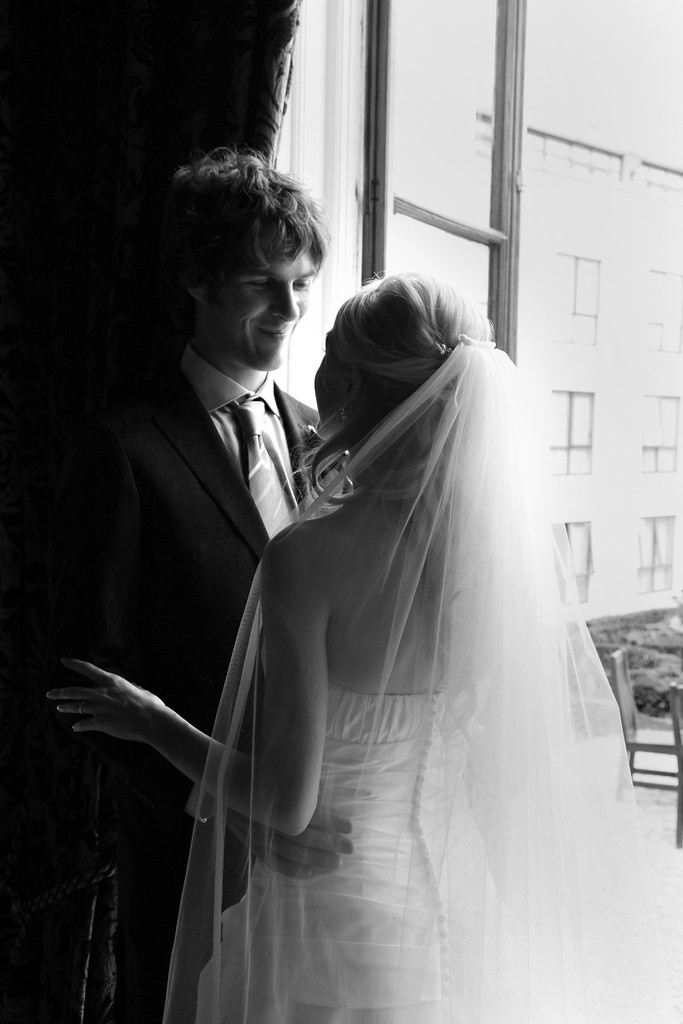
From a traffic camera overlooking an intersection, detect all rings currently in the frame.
[78,699,86,715]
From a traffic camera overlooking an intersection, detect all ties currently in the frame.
[228,396,306,538]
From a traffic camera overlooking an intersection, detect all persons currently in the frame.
[46,275,683,1024]
[47,149,353,1023]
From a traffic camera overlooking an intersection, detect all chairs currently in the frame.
[669,681,683,849]
[611,646,677,790]
